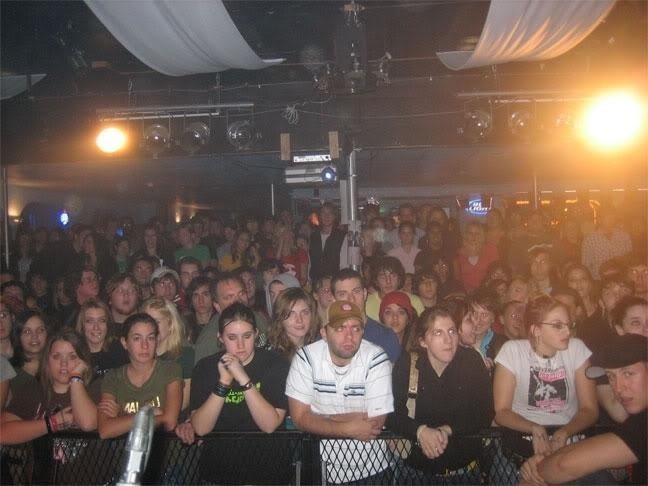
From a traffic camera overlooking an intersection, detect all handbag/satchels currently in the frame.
[385,398,416,459]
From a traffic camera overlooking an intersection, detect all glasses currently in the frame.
[81,276,102,283]
[540,322,576,329]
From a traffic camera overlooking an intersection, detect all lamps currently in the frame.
[506,98,536,140]
[457,99,497,143]
[139,113,173,154]
[225,108,260,151]
[314,4,392,94]
[180,112,214,154]
[97,114,129,155]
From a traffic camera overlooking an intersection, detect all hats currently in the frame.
[323,301,363,329]
[585,332,648,379]
[150,266,180,286]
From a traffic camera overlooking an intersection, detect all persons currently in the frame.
[1,201,648,485]
